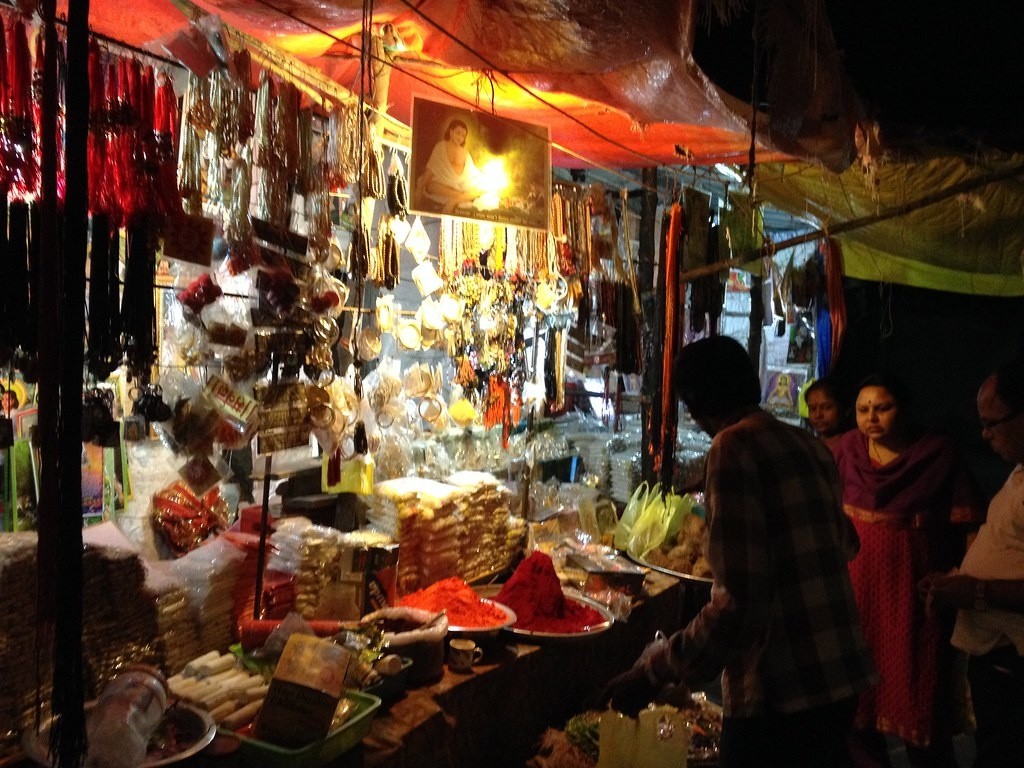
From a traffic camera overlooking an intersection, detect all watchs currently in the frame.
[973,580,989,611]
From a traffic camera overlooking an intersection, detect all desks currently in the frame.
[167,566,681,768]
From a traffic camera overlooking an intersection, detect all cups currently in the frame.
[448,639,484,674]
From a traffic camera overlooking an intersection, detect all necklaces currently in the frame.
[871,439,881,463]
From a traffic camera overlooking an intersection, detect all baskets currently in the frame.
[227,642,414,717]
[214,685,382,768]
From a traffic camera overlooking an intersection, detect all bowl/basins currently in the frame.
[23,698,217,768]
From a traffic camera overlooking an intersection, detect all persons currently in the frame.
[916,355,1024,768]
[831,374,959,768]
[635,336,870,768]
[806,377,852,452]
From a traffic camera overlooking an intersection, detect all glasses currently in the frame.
[976,410,1022,432]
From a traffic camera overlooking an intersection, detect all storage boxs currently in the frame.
[564,552,645,597]
[216,687,382,768]
